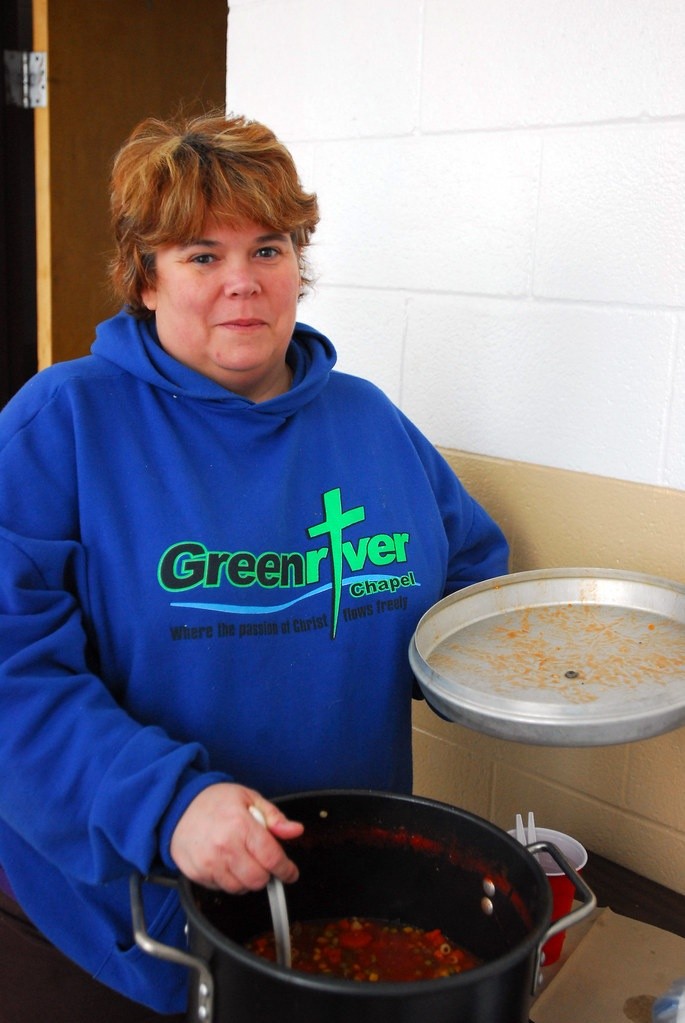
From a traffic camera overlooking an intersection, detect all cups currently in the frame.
[507,828,588,968]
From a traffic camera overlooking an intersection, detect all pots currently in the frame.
[131,788,598,1023]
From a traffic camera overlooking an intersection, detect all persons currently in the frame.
[0,111,510,1023]
